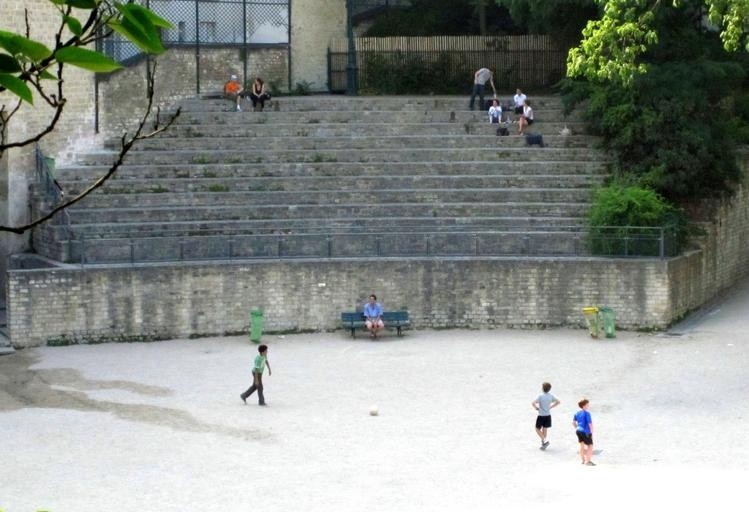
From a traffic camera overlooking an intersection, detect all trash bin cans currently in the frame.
[581,306,616,338]
[42,157,55,181]
[249,311,264,343]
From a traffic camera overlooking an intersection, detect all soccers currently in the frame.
[369,405,379,415]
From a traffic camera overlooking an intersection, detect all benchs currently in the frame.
[342,310,412,339]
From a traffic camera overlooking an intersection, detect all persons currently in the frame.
[363,294,384,337]
[252,77,266,113]
[488,99,503,124]
[572,400,596,465]
[532,383,560,450]
[518,100,534,136]
[513,88,526,114]
[469,67,497,111]
[224,75,244,112]
[241,345,271,406]
